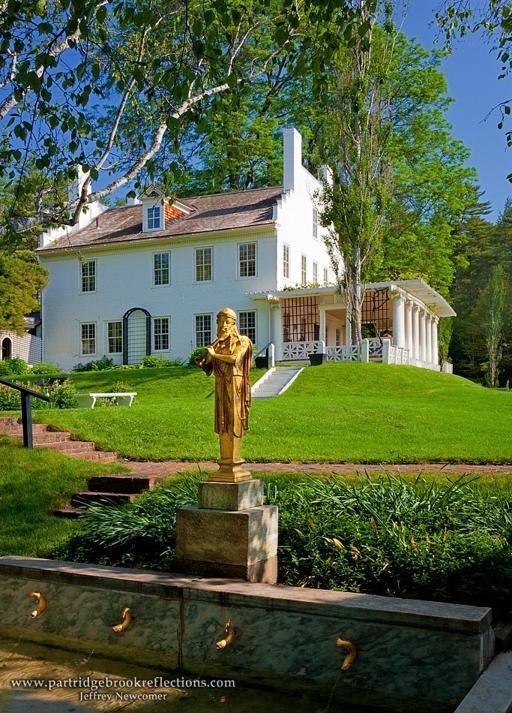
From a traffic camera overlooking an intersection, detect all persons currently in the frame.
[382,330,392,337]
[196,308,253,465]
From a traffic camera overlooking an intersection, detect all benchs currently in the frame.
[89,392,137,409]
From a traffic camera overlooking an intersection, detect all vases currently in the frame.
[255,357,268,368]
[308,354,328,366]
